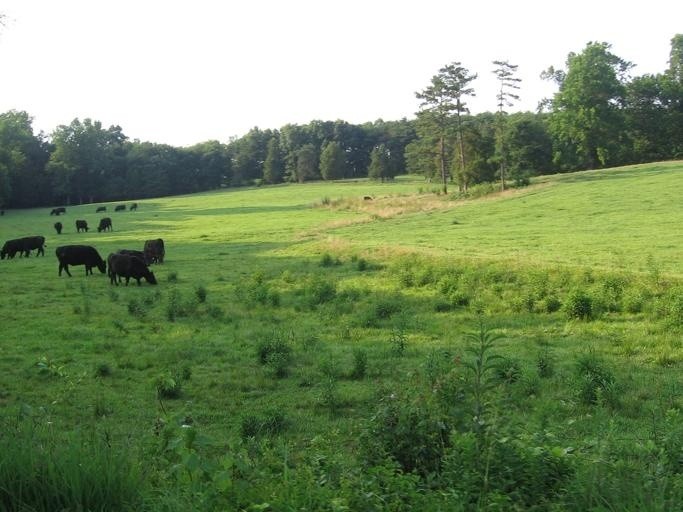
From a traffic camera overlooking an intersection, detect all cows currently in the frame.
[55,245,107,277]
[0,236,47,260]
[107,238,166,286]
[96,206,106,213]
[129,203,137,211]
[54,222,63,234]
[97,217,113,233]
[76,219,90,233]
[115,205,126,212]
[51,206,65,215]
